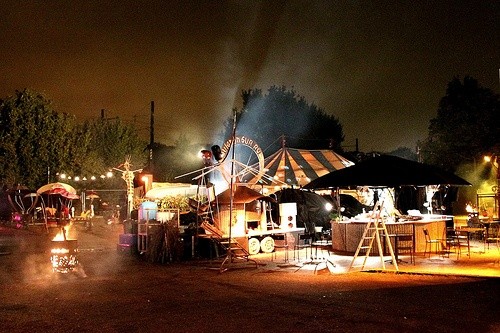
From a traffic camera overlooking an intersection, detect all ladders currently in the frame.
[347,200,400,273]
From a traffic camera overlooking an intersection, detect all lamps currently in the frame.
[484,152,495,162]
[211,145,223,161]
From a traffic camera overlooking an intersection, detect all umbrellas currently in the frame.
[2,177,101,217]
[226,135,356,189]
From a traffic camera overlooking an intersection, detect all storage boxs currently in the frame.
[119,233,137,246]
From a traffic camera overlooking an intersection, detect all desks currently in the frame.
[455,228,486,260]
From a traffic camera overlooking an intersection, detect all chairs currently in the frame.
[381,222,416,267]
[480,220,499,253]
[293,245,311,262]
[440,227,462,258]
[423,228,440,258]
[271,245,289,263]
